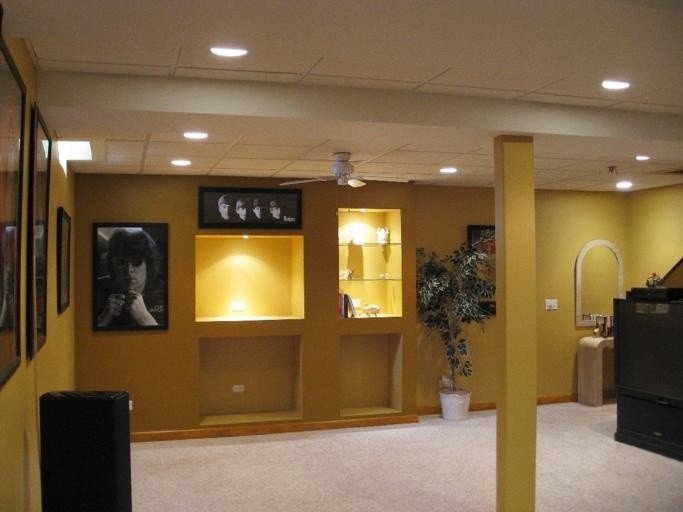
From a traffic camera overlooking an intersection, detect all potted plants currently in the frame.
[412,230,496,420]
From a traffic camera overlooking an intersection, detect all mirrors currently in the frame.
[574,238,624,328]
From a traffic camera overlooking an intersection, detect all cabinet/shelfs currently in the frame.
[338,208,404,427]
[195,234,306,427]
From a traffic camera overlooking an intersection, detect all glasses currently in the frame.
[107,254,145,269]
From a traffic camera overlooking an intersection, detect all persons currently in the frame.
[217,194,235,221]
[234,196,253,224]
[94,228,166,326]
[251,198,270,223]
[266,199,286,223]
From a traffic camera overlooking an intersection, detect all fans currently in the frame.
[279,151,409,189]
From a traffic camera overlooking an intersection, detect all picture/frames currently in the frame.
[198,185,302,230]
[92,222,168,331]
[466,225,497,315]
[0,35,71,390]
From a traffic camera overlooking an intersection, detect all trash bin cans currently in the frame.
[40,391,131,512]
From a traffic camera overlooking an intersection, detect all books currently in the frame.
[348,294,357,318]
[338,293,344,319]
[342,294,350,318]
[596,313,614,338]
